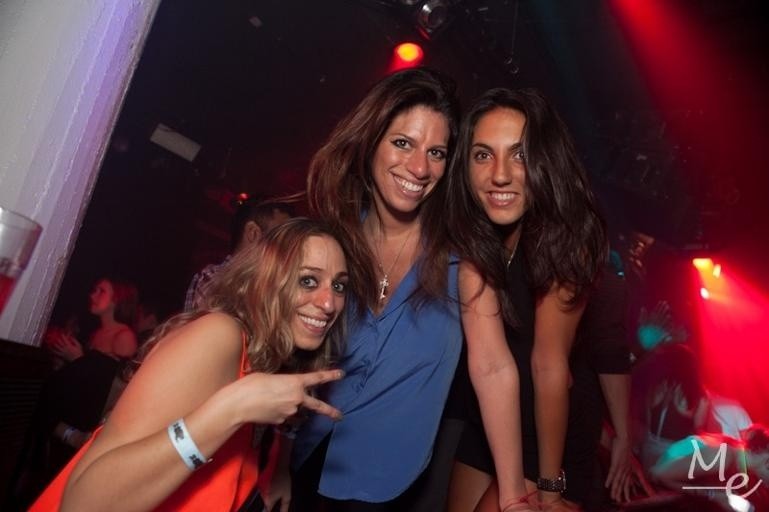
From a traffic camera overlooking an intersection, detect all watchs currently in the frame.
[536,467,567,493]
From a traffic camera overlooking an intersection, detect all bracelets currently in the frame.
[168,417,209,472]
[498,491,536,512]
[272,416,305,440]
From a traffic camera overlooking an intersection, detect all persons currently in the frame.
[630,298,676,363]
[257,67,534,512]
[54,274,140,373]
[444,84,611,503]
[24,218,360,511]
[182,194,298,311]
[562,249,658,503]
[626,233,706,418]
[132,296,164,347]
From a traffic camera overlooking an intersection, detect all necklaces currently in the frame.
[506,224,522,268]
[365,211,413,301]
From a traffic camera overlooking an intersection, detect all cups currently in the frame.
[0,206,43,320]
